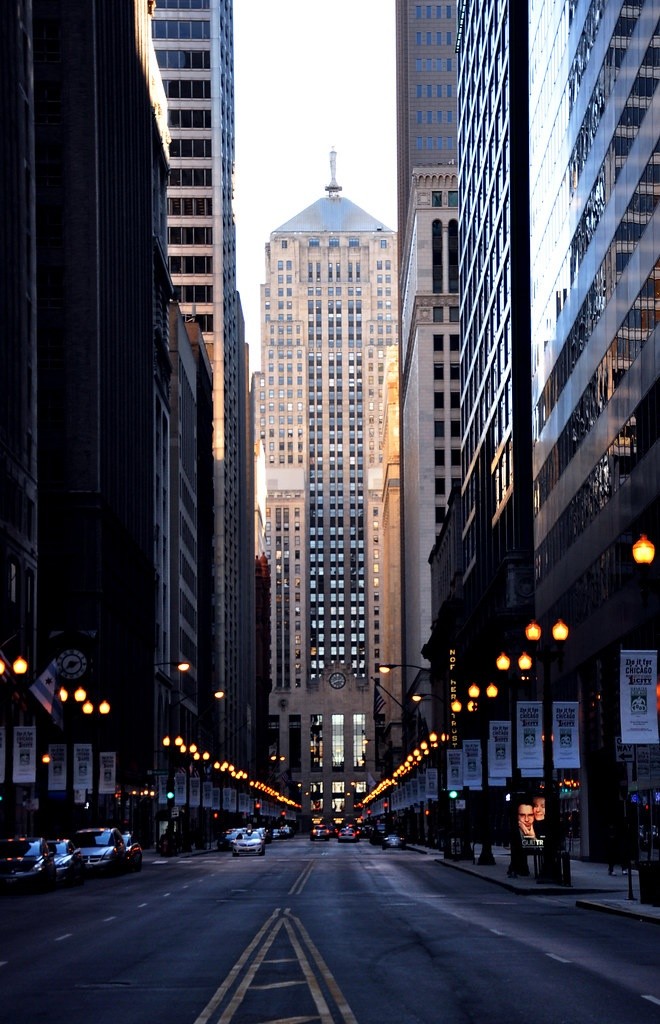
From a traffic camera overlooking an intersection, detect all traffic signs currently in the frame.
[615,737,635,762]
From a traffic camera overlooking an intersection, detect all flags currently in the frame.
[29,658,64,730]
[373,685,386,724]
[281,767,292,784]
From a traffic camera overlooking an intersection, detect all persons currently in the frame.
[532,793,545,821]
[606,816,628,876]
[519,802,540,846]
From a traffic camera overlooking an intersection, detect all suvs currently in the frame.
[71,827,125,876]
[310,825,330,841]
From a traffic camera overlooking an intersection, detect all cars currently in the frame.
[47,840,86,888]
[218,825,295,857]
[328,824,406,850]
[0,836,57,893]
[122,831,143,872]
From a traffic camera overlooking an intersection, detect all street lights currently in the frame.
[452,699,478,861]
[162,735,307,852]
[57,685,87,832]
[1,656,28,835]
[469,682,499,865]
[496,651,532,874]
[524,617,571,883]
[379,663,450,858]
[121,658,190,831]
[168,684,225,856]
[361,731,450,851]
[83,697,111,827]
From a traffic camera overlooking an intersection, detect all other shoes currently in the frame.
[609,871,617,876]
[622,870,627,875]
